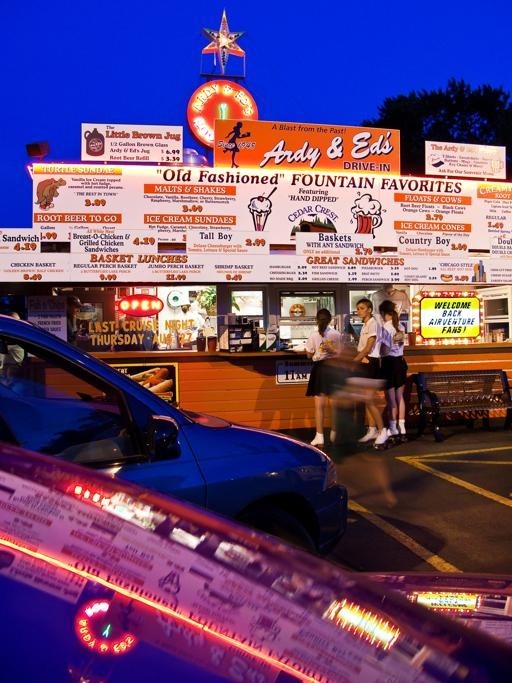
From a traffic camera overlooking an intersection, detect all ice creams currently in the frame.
[351,193,382,234]
[247,195,271,231]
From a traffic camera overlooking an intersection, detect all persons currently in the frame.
[379,300,408,435]
[352,299,392,444]
[306,309,343,445]
[222,120,253,167]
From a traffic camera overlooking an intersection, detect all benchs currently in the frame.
[413,370,512,442]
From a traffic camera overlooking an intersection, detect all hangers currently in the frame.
[372,284,407,296]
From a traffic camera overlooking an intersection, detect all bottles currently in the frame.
[85,128,105,157]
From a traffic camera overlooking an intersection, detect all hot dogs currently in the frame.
[441,274,453,282]
[129,368,174,393]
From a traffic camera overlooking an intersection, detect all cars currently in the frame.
[0,315,347,557]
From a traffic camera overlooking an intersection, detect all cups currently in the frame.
[196,336,216,353]
[354,213,381,238]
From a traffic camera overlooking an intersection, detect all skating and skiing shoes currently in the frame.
[311,432,324,449]
[357,419,408,450]
[330,431,336,445]
